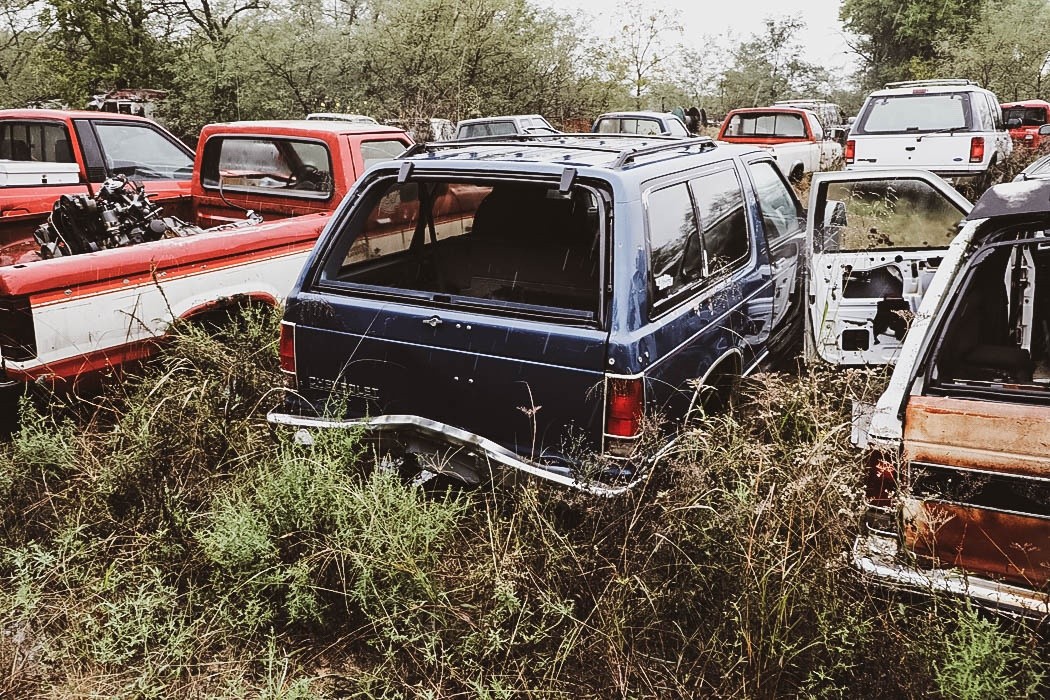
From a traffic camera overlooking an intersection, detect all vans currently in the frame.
[1000,99,1050,150]
[844,80,1022,192]
[769,99,850,138]
[385,119,457,143]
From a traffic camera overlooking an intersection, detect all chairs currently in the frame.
[56,139,71,163]
[0,138,28,160]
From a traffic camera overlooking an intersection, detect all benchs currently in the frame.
[467,190,601,297]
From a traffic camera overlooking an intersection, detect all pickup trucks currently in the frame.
[593,111,700,137]
[717,108,843,182]
[0,121,491,396]
[0,110,264,217]
[223,113,379,159]
[411,112,579,143]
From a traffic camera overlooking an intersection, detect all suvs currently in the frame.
[265,131,848,506]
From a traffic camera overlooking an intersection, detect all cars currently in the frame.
[805,156,1050,626]
